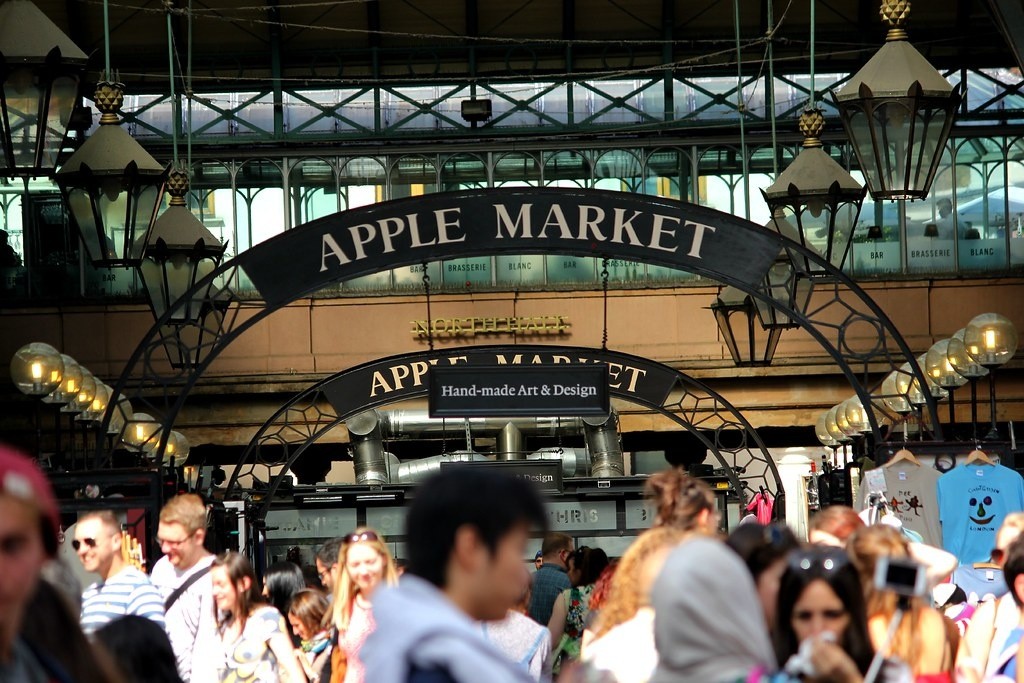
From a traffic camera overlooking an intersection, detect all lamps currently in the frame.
[51,0,175,272]
[141,1,243,374]
[461,99,493,123]
[710,0,783,366]
[60,106,93,132]
[718,470,729,489]
[814,312,1019,468]
[736,0,825,331]
[12,343,191,472]
[130,1,231,329]
[826,0,968,203]
[759,1,867,280]
[248,480,267,501]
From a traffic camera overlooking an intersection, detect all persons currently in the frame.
[0,446,1024,683]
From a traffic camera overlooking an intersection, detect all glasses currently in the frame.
[348,531,379,543]
[154,532,195,548]
[989,548,1006,563]
[0,530,48,555]
[70,532,114,550]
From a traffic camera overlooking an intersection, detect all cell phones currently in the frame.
[875,556,926,597]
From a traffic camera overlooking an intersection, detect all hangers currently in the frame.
[965,438,996,468]
[882,443,925,471]
